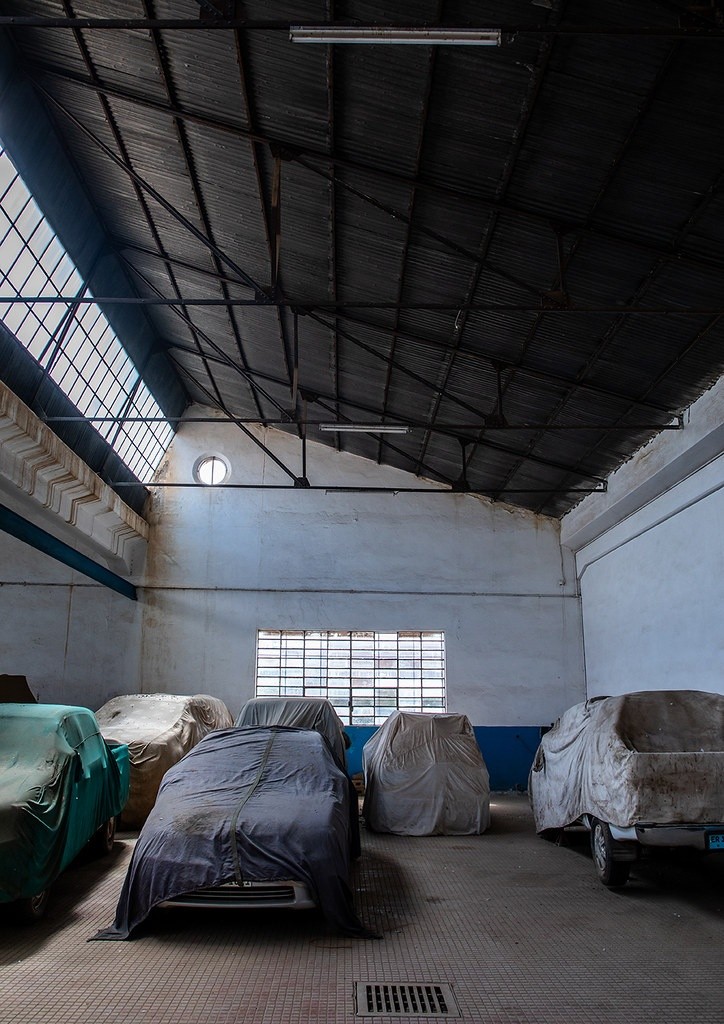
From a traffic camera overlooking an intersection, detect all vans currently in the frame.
[232,697,351,768]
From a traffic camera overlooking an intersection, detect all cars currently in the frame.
[0,703,129,918]
[127,725,359,910]
[93,692,232,821]
[363,710,490,834]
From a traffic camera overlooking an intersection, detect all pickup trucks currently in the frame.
[527,690,724,885]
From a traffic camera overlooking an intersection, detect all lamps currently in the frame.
[288,25,501,47]
[319,423,413,434]
